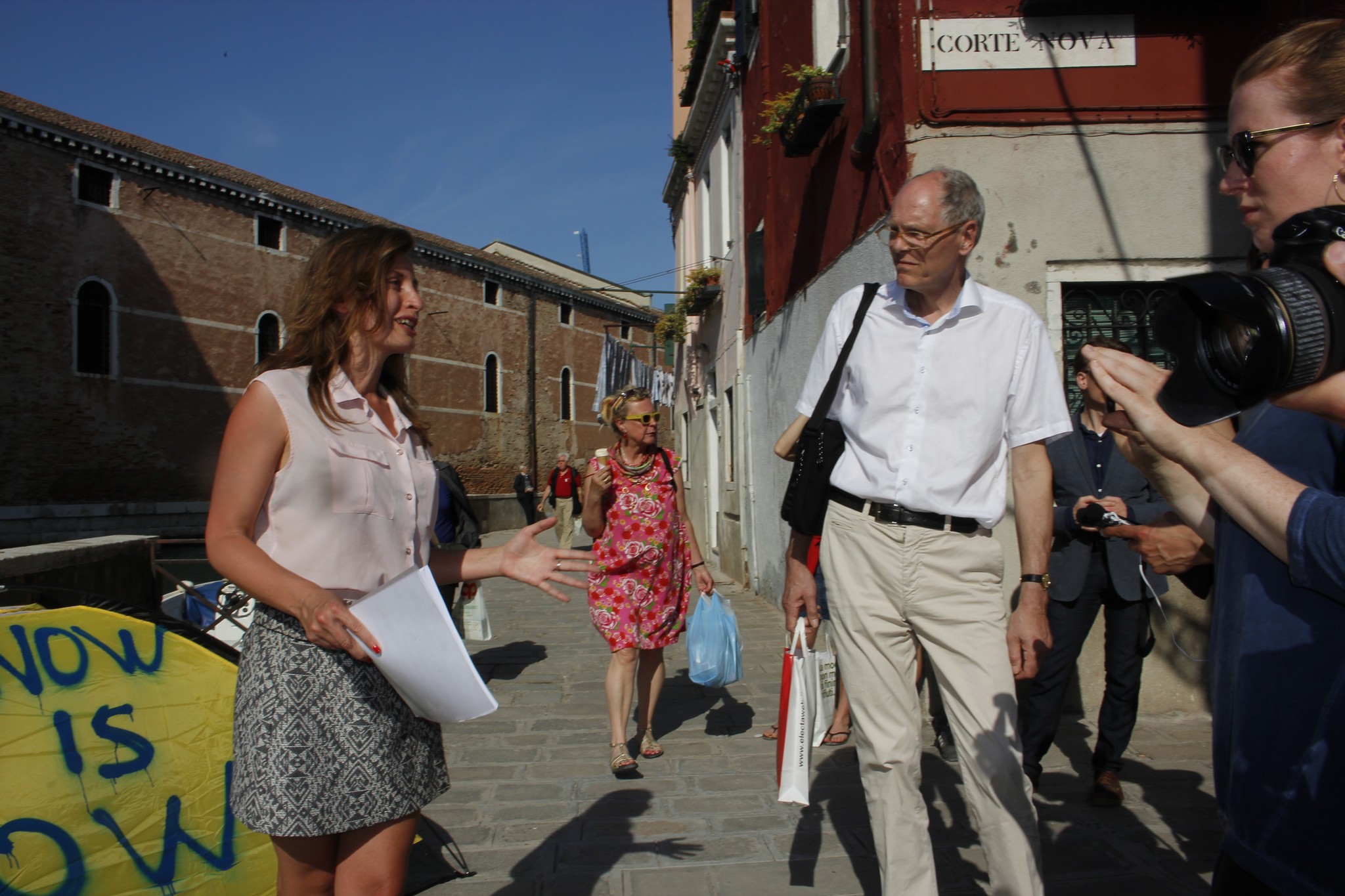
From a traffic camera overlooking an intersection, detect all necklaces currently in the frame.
[620,447,628,463]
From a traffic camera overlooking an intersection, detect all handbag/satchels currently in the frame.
[686,589,745,688]
[780,284,876,533]
[776,616,816,806]
[813,632,837,747]
[700,589,744,651]
[453,578,494,641]
[572,517,583,534]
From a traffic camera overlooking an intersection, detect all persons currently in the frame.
[1019,337,1174,806]
[206,219,605,896]
[582,385,715,773]
[1080,18,1345,896]
[782,165,1073,896]
[761,415,852,746]
[513,465,536,526]
[536,452,584,550]
[432,459,481,616]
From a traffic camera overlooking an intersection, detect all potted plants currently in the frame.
[653,264,726,347]
[751,64,837,148]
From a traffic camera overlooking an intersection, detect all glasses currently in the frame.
[613,387,646,410]
[875,221,968,249]
[1217,116,1345,177]
[621,412,660,424]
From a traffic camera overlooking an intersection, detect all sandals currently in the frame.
[609,742,638,772]
[636,728,664,758]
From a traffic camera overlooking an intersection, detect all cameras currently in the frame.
[1152,202,1345,428]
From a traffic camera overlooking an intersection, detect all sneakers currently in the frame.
[936,734,958,761]
[1094,768,1122,805]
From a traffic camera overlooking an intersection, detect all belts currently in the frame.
[826,484,979,533]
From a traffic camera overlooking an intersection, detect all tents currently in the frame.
[0,599,471,896]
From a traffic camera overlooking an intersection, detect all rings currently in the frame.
[700,590,702,592]
[603,479,608,484]
[557,560,561,570]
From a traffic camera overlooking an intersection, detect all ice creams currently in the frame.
[595,448,609,470]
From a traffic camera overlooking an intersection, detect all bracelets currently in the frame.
[541,501,544,502]
[692,561,704,569]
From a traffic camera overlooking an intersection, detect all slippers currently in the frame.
[824,729,850,745]
[763,725,779,740]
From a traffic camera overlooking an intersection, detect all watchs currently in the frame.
[1020,573,1052,590]
